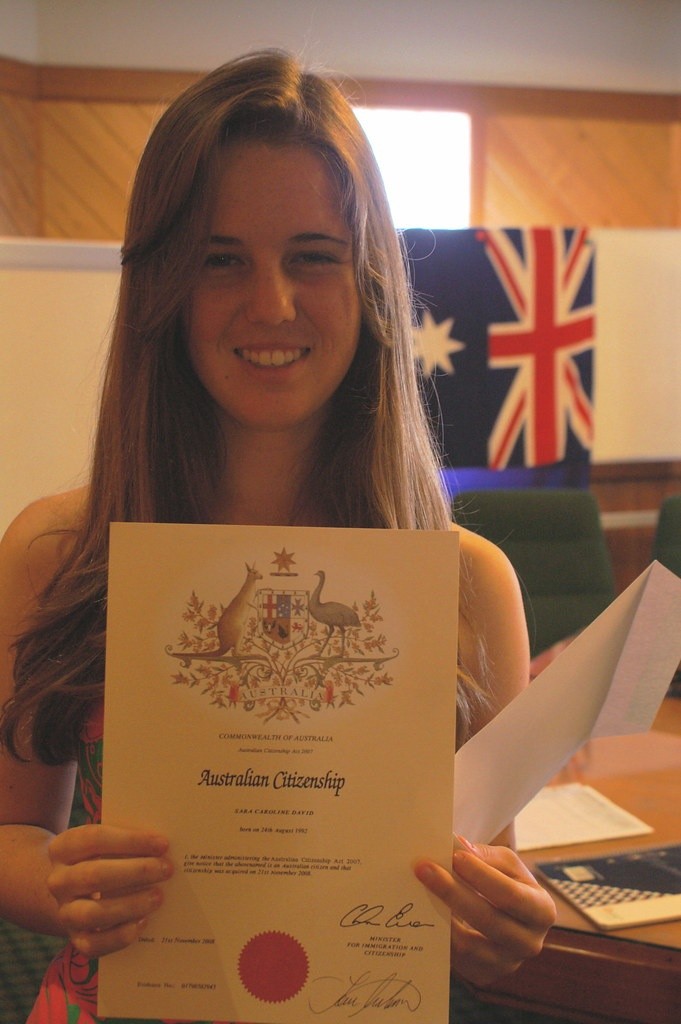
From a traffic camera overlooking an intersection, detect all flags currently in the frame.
[399,223,597,505]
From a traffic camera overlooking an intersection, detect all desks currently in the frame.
[477,697,681,1024]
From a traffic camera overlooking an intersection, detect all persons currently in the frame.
[1,48,556,1024]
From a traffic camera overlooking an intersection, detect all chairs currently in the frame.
[453,490,613,658]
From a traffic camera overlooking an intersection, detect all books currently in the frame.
[534,844,681,928]
